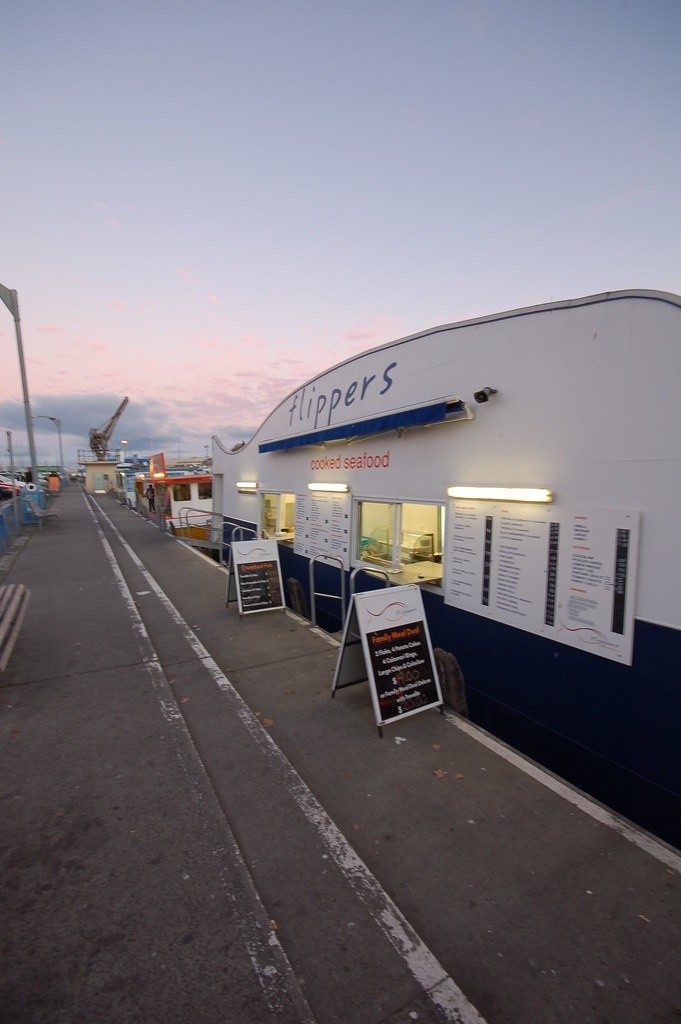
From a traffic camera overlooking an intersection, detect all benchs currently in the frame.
[0,584,33,672]
[25,495,61,532]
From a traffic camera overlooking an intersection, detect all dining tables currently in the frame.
[252,527,295,544]
[364,560,443,586]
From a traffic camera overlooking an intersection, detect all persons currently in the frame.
[145,484,156,514]
[25,467,32,483]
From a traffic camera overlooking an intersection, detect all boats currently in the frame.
[117,462,212,556]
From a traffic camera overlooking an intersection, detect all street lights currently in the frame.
[39,415,65,486]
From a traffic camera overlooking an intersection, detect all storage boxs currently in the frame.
[368,525,435,565]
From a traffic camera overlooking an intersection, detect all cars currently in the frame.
[0,474,27,500]
